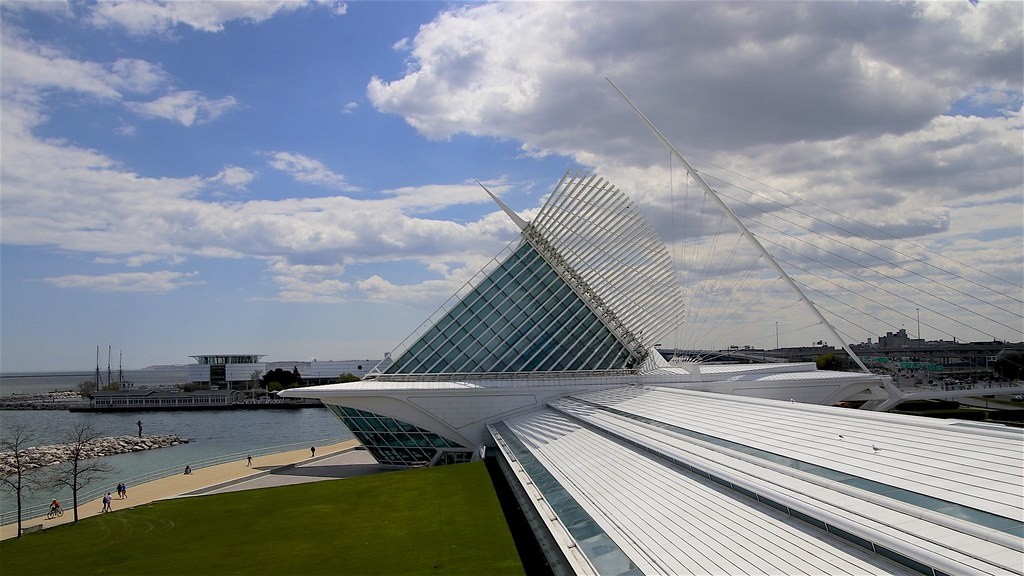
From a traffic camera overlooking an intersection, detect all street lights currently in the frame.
[916,308,921,338]
[775,322,778,350]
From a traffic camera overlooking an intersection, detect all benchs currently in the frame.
[22,523,44,535]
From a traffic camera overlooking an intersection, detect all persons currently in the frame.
[121,483,127,499]
[50,499,60,516]
[102,493,108,512]
[247,455,253,466]
[311,446,315,456]
[117,483,122,498]
[106,492,113,512]
[184,465,191,474]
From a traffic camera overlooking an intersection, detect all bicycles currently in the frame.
[46,506,64,519]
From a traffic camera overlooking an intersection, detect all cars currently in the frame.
[1011,394,1024,401]
